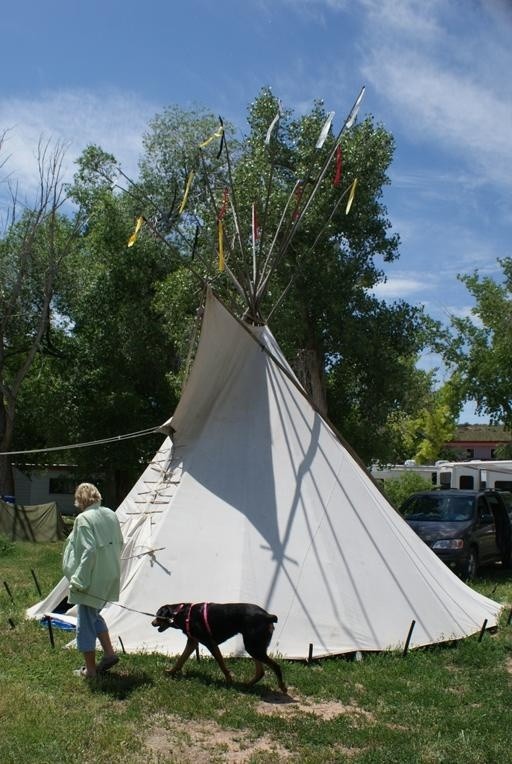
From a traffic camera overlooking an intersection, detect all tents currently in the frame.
[20,284,506,665]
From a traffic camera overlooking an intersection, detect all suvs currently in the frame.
[390,483,512,584]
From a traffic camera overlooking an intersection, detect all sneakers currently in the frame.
[71,665,99,679]
[97,654,120,671]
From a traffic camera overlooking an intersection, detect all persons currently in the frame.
[62,479,125,679]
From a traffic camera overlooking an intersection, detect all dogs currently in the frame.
[151,602,288,695]
